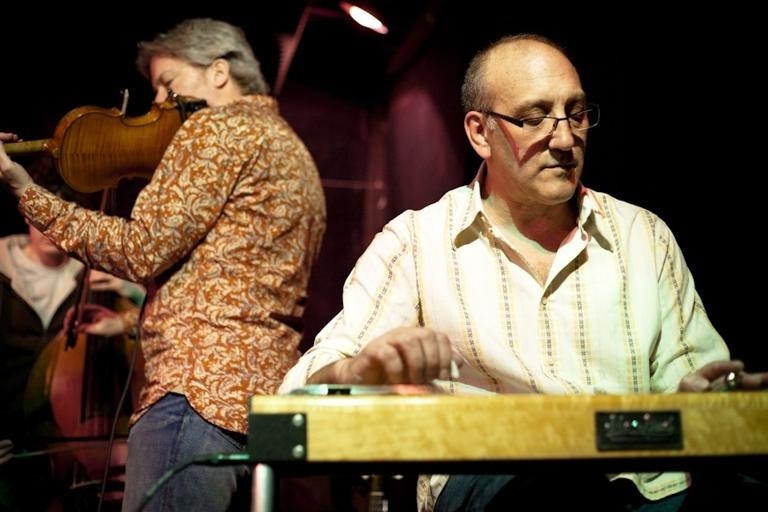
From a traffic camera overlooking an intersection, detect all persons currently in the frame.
[277,32,767,510]
[2,15,329,511]
[1,154,144,508]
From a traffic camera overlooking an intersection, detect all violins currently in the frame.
[1,96,209,192]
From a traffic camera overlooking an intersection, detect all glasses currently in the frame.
[487,100,601,138]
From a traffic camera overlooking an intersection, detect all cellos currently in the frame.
[23,187,146,486]
